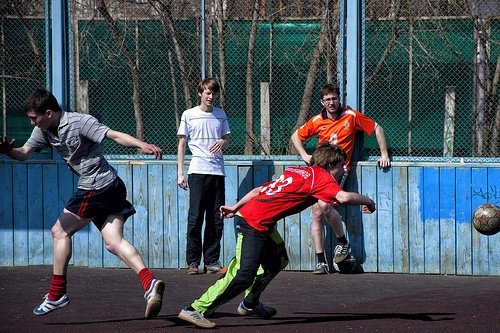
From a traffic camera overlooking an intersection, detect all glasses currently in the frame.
[323,97,338,103]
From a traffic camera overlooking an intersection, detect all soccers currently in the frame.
[473,204,500,235]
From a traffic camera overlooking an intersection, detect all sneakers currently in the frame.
[33,293,70,316]
[186,263,199,276]
[333,242,350,265]
[178,308,216,328]
[237,302,278,318]
[203,260,229,274]
[144,278,165,320]
[312,263,330,275]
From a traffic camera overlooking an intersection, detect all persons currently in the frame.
[178,78,232,275]
[291,84,391,274]
[0,89,165,320]
[178,144,375,328]
